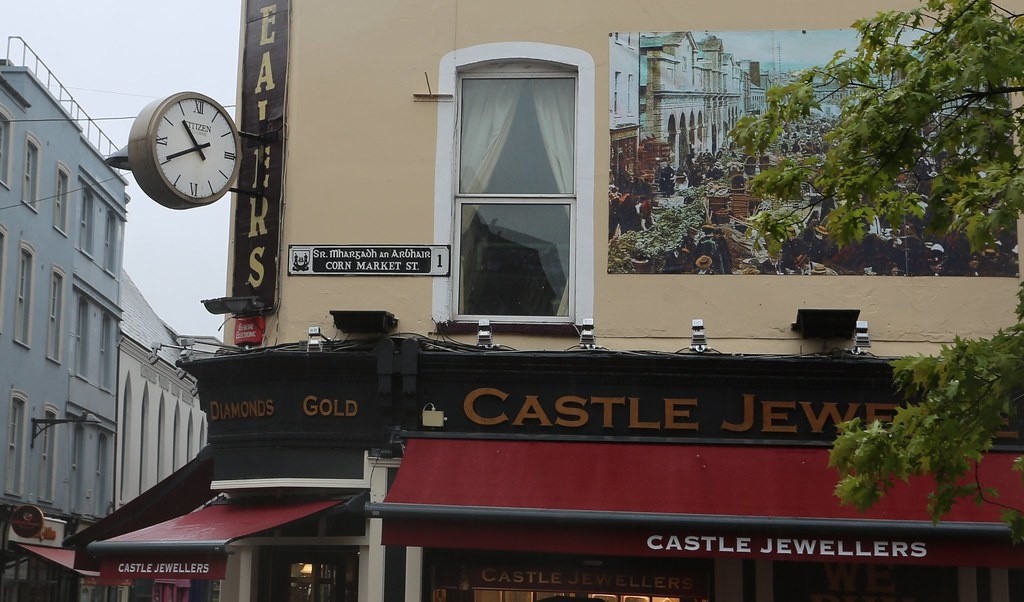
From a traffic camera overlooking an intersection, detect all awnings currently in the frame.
[59,443,213,572]
[16,543,133,586]
[86,493,345,582]
[364,432,1024,572]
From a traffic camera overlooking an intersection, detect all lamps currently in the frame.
[330,308,398,338]
[478,319,493,346]
[691,319,709,347]
[29,410,102,451]
[307,328,330,353]
[579,319,596,345]
[147,338,218,397]
[855,321,872,349]
[198,296,270,317]
[791,305,860,340]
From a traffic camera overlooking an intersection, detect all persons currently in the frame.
[609,110,1021,278]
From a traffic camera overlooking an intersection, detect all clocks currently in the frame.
[108,90,242,211]
